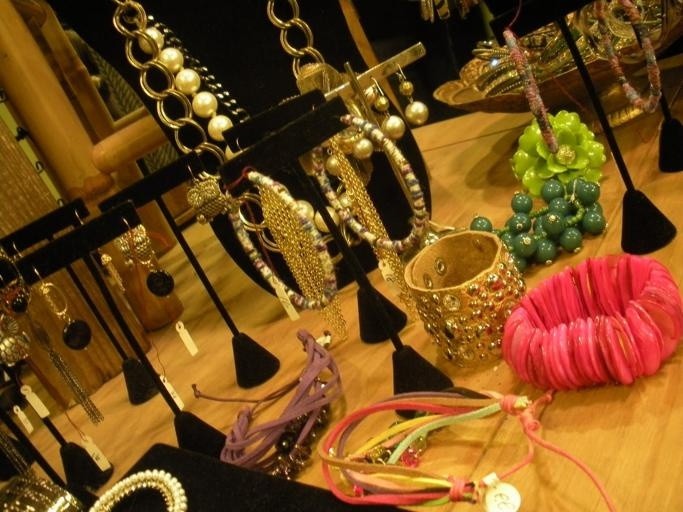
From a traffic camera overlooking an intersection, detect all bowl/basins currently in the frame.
[433,0,683,136]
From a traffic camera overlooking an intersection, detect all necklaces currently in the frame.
[113,0,330,191]
[88,469,189,512]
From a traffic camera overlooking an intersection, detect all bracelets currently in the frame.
[403,230,529,368]
[311,114,428,252]
[596,0,662,113]
[504,28,558,153]
[227,171,338,310]
